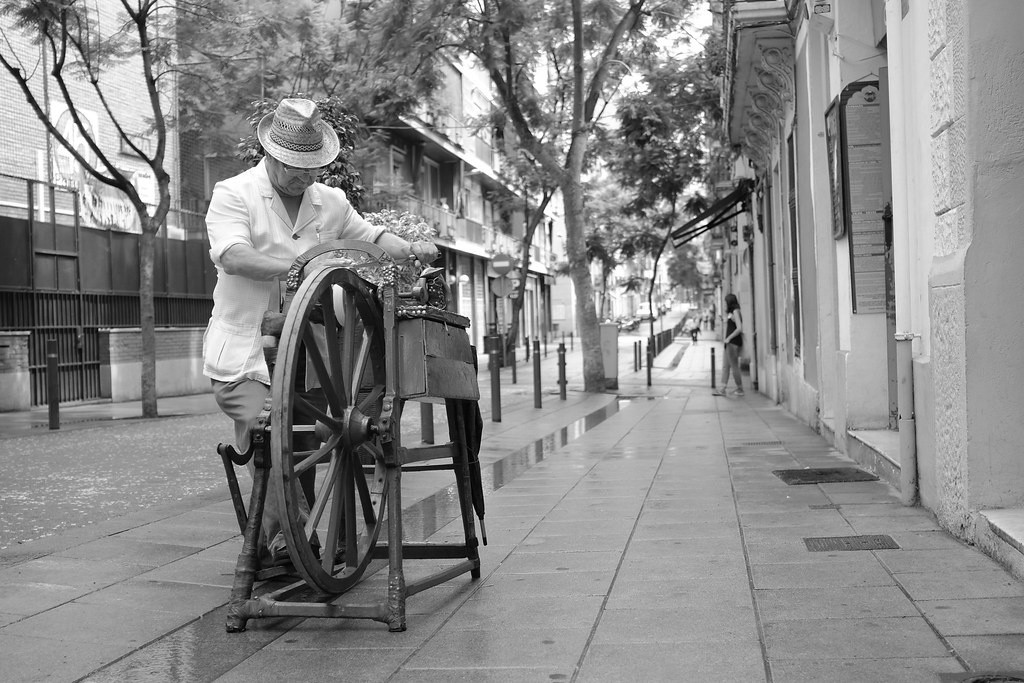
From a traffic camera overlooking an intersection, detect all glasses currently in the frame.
[280,163,328,177]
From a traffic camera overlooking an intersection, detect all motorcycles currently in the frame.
[616,314,641,332]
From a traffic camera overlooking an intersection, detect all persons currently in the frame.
[688,316,702,346]
[709,309,715,331]
[702,309,708,330]
[711,293,746,397]
[201,98,439,578]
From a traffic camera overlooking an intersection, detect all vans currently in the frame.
[636,302,658,321]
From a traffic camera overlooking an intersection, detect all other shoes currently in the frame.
[255,551,293,583]
[712,390,726,397]
[729,389,745,396]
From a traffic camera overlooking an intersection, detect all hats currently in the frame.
[257,98,341,169]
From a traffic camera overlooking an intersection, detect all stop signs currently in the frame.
[492,254,512,276]
